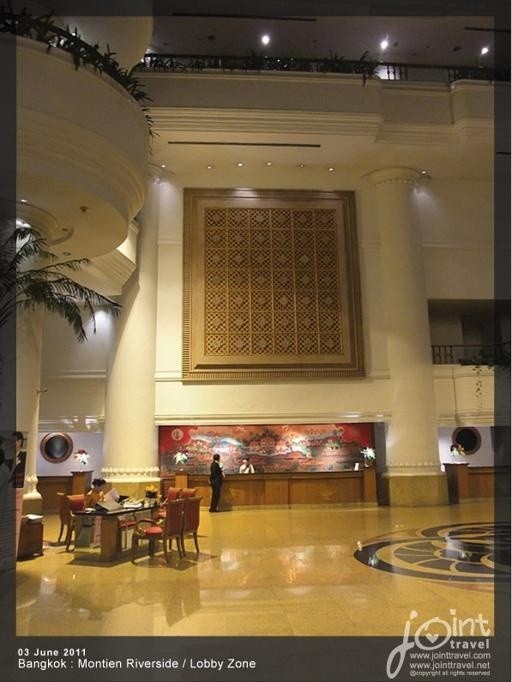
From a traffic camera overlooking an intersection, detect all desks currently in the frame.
[70,469,96,495]
[443,462,471,505]
[172,468,192,488]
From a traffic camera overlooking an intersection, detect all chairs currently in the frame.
[16,485,204,565]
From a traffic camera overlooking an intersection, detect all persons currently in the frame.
[82,478,107,511]
[238,456,257,474]
[0,435,22,487]
[7,431,27,489]
[208,453,224,511]
